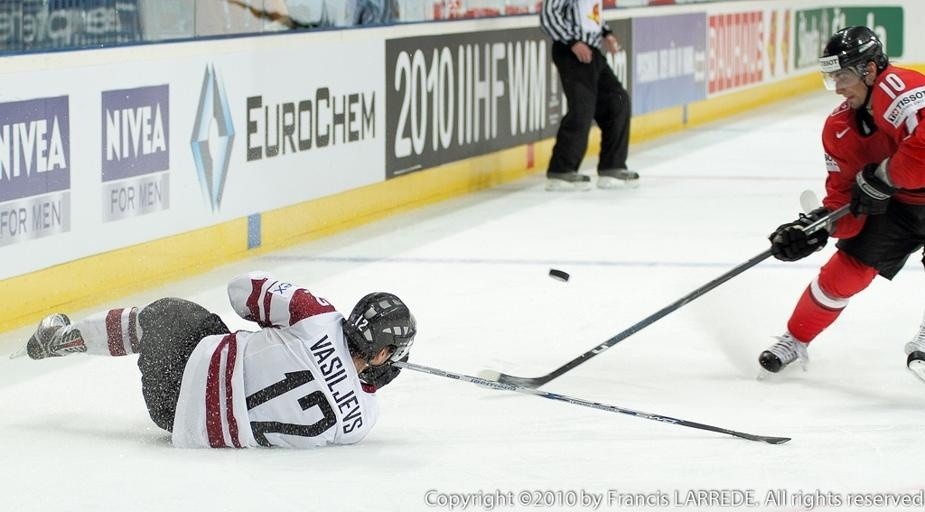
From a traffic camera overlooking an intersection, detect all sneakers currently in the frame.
[547,170,591,183]
[759,334,807,372]
[598,166,639,180]
[906,351,925,380]
[905,317,925,352]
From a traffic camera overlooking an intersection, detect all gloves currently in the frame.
[360,354,404,393]
[850,163,896,218]
[769,205,838,262]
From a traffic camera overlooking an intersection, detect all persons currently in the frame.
[25,273,417,452]
[535,1,641,198]
[756,24,925,389]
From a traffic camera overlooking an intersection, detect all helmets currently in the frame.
[817,25,889,92]
[341,292,418,362]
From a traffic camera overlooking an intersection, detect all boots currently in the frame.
[25,310,87,361]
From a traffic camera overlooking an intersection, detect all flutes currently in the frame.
[389,360,791,445]
